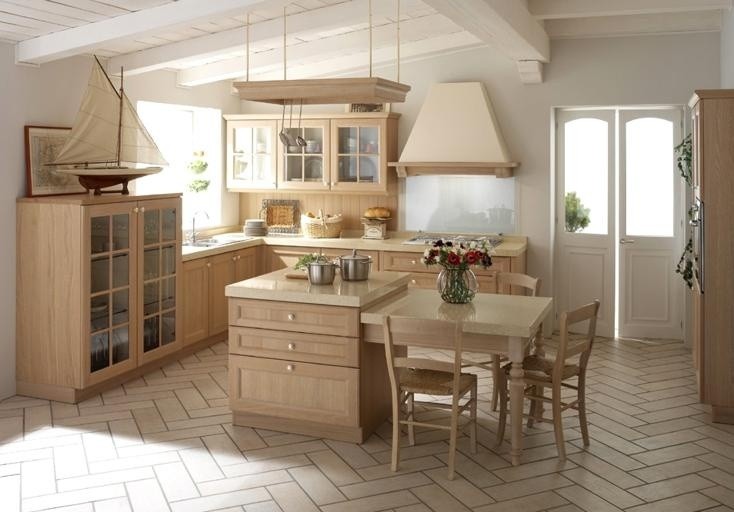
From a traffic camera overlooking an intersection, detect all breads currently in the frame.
[364,207,390,219]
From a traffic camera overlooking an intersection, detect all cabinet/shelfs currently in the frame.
[178,228,530,364]
[222,112,402,195]
[16,193,182,404]
[225,264,412,444]
[688,90,734,423]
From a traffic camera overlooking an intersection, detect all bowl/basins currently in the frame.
[305,140,316,153]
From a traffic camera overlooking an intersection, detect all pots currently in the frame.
[338,248,373,282]
[306,255,335,285]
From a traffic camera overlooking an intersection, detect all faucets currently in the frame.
[189,209,209,243]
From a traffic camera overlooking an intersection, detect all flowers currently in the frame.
[420,239,496,303]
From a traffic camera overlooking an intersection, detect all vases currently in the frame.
[437,270,479,303]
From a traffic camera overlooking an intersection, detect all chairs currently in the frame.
[491,270,544,428]
[496,298,601,463]
[383,313,477,481]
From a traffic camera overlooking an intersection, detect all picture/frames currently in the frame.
[260,199,301,234]
[24,125,89,197]
[346,104,390,112]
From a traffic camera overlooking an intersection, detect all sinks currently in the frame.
[181,236,253,247]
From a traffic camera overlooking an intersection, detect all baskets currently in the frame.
[301,208,342,238]
